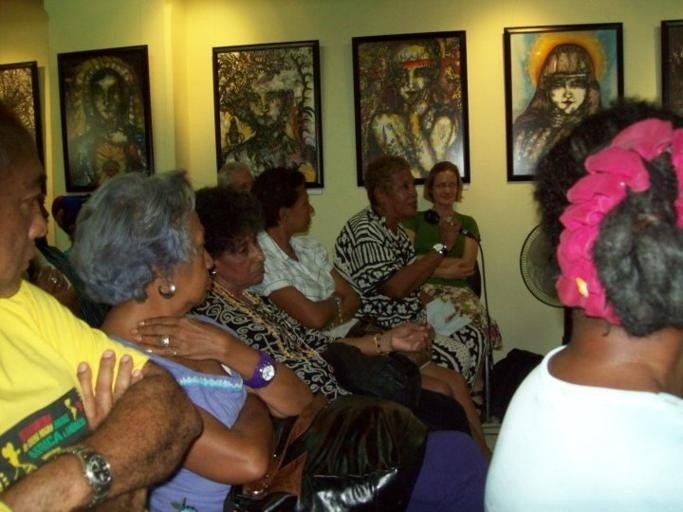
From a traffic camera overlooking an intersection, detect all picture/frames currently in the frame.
[57,45,154,192]
[0,60,47,195]
[659,19,683,123]
[351,30,470,187]
[503,22,624,180]
[212,39,324,189]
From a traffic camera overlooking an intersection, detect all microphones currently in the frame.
[424,209,476,239]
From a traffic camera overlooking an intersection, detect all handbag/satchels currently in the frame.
[219,391,430,512]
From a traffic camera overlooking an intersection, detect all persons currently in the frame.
[367,39,459,180]
[509,44,602,176]
[220,71,317,183]
[71,68,149,192]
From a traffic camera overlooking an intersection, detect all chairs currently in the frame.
[462,260,492,422]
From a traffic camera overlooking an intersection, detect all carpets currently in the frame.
[483,347,544,424]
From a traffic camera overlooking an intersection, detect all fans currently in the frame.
[520,222,574,345]
